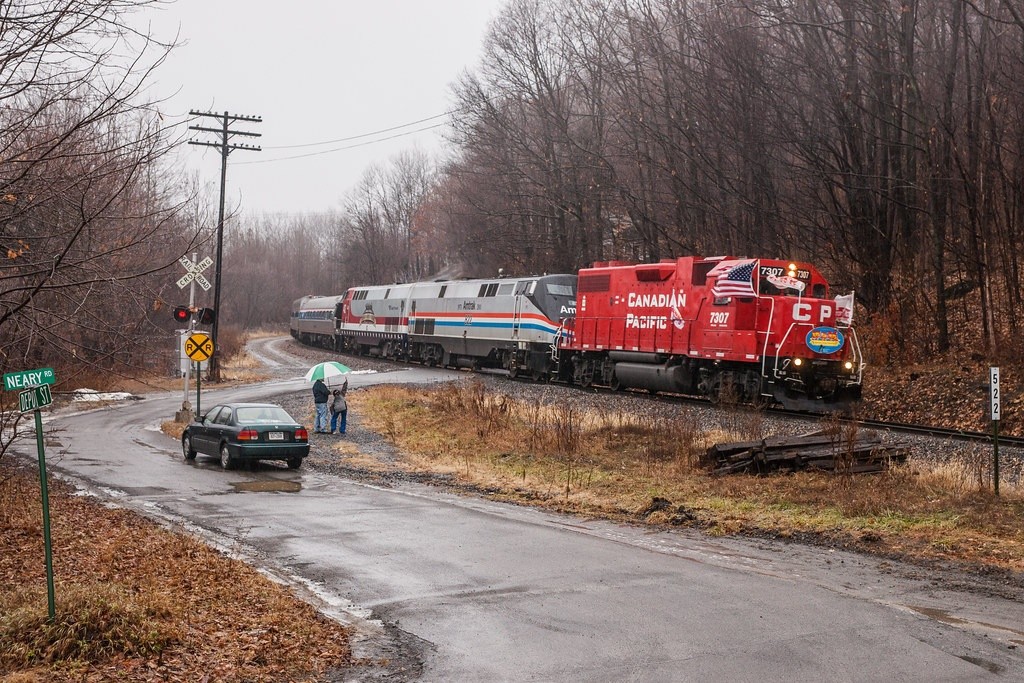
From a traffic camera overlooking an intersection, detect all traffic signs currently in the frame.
[18,383,53,414]
[3,367,55,391]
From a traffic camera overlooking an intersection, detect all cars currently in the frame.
[182,403,310,469]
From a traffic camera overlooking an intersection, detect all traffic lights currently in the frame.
[198,308,215,325]
[174,305,190,322]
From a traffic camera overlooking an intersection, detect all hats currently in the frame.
[333,390,340,395]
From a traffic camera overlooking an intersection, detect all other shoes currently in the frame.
[314,429,347,435]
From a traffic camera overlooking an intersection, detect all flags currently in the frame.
[833,293,856,325]
[712,258,760,298]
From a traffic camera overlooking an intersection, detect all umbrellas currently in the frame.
[305,360,350,389]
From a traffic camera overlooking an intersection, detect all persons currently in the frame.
[312,378,331,434]
[330,376,348,434]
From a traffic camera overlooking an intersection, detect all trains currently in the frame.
[291,256,867,414]
